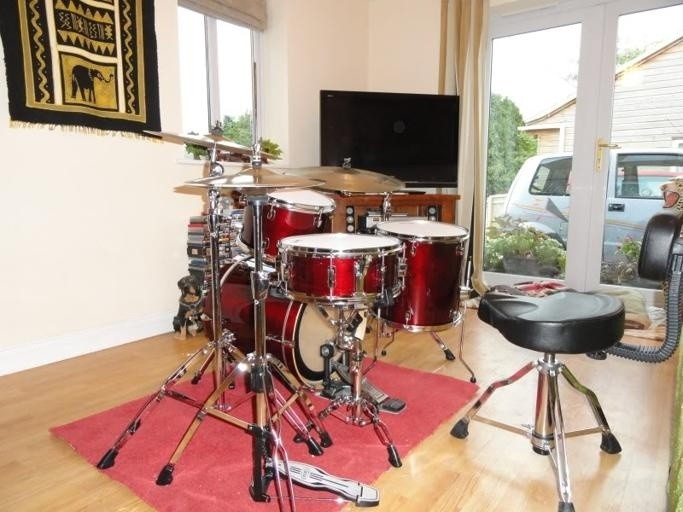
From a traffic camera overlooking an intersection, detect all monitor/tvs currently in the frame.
[320,89,460,194]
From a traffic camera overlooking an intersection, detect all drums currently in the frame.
[203,264,354,392]
[276,232,408,307]
[370,220,470,333]
[235,188,337,263]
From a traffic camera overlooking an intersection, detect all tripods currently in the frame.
[96,153,323,470]
[155,203,332,501]
[294,307,402,467]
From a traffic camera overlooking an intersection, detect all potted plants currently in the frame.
[484,215,547,275]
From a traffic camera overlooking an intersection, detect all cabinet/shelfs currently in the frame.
[321,191,461,234]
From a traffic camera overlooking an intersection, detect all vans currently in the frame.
[495,142,682,291]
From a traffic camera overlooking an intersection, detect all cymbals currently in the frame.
[143,129,283,160]
[283,166,406,193]
[184,168,327,189]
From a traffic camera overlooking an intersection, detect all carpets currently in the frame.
[50,356,479,512]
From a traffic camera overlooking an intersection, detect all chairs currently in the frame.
[450,214,683,455]
